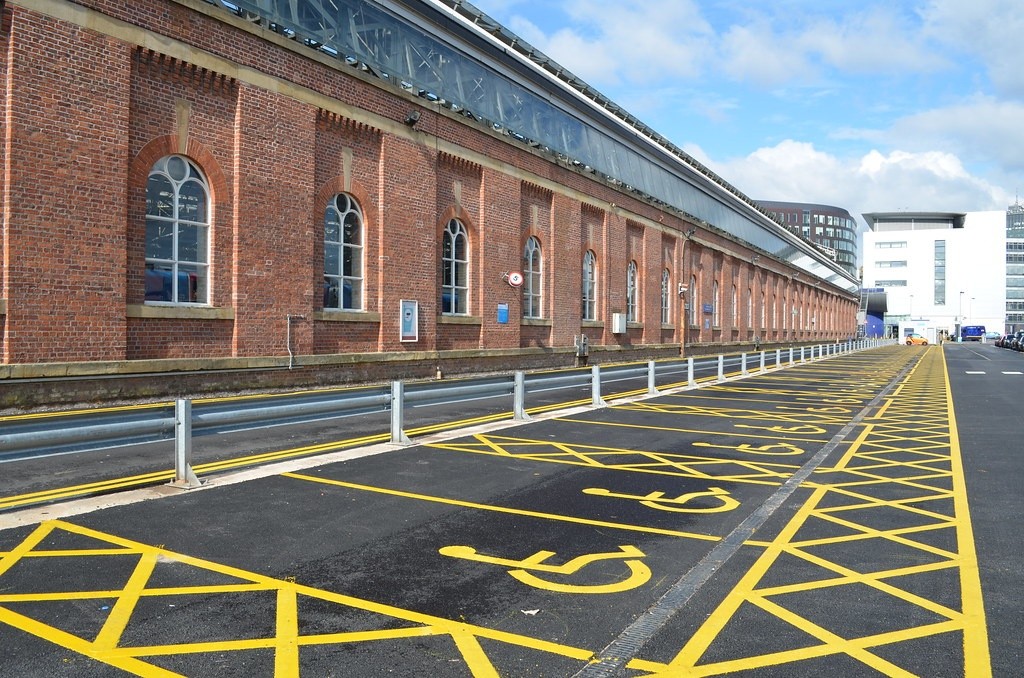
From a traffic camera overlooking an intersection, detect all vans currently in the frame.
[952,326,986,341]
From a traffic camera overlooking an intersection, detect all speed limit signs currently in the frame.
[508,272,524,287]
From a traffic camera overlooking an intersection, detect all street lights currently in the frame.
[959,292,964,324]
[970,298,974,325]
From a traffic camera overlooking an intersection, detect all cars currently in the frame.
[986,332,1002,340]
[995,331,1024,351]
[906,333,928,346]
